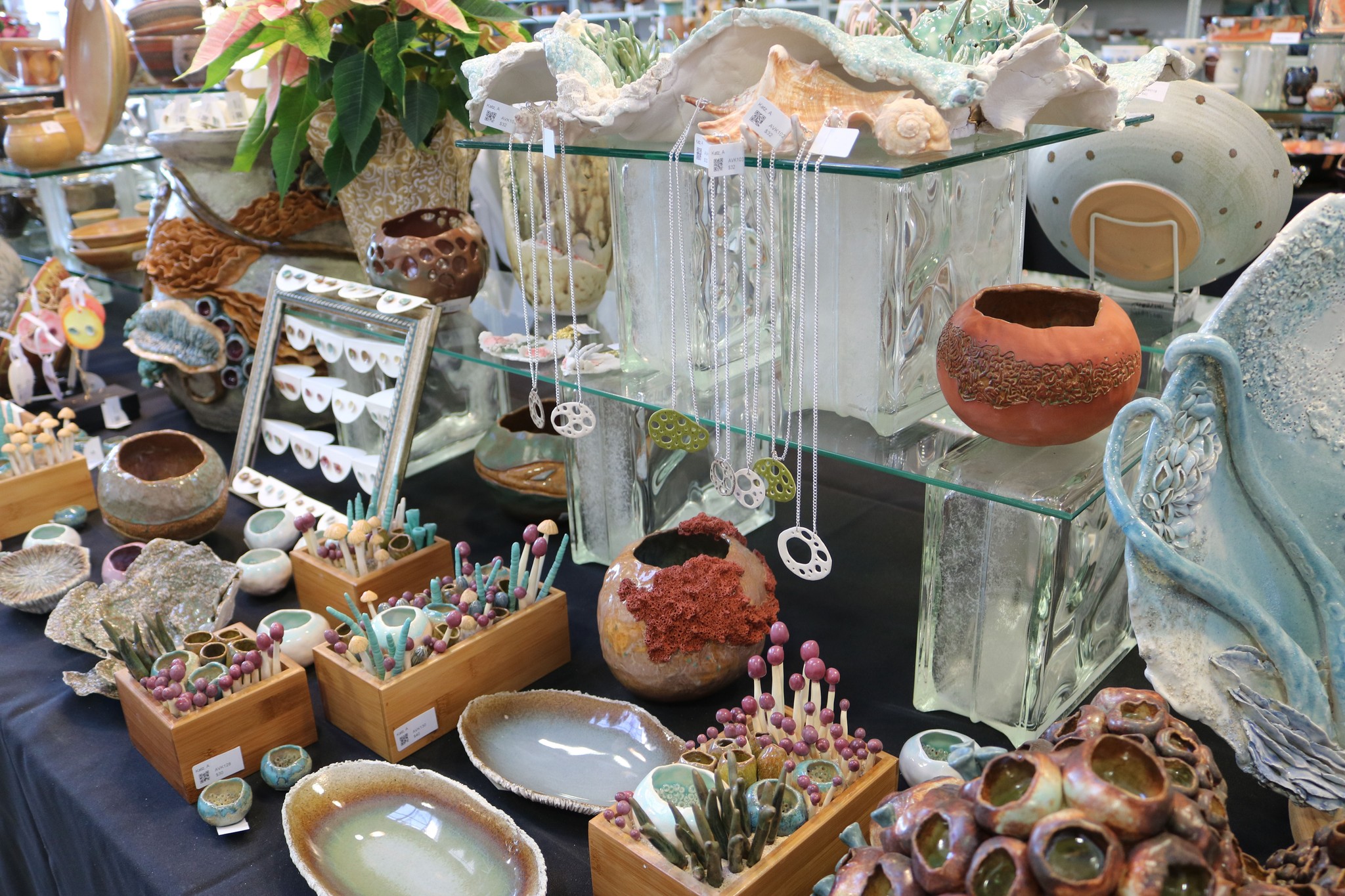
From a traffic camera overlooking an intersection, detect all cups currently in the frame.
[12,45,63,87]
[0,186,28,237]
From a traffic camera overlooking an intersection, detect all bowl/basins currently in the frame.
[126,0,206,37]
[11,184,45,226]
[1024,75,1295,292]
[171,34,206,89]
[70,206,122,228]
[67,217,151,248]
[126,34,187,89]
[0,255,71,380]
[132,198,153,216]
[64,0,132,157]
[69,239,149,274]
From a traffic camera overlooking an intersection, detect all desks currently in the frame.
[0,273,1294,896]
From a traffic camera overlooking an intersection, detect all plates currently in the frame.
[278,759,550,896]
[456,686,690,817]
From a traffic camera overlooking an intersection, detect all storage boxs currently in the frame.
[588,705,899,896]
[312,567,570,764]
[0,442,99,541]
[114,622,318,804]
[289,534,455,628]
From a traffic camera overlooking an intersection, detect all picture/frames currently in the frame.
[227,270,442,529]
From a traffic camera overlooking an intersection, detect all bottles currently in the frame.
[1283,67,1341,111]
[597,513,780,702]
[3,105,86,172]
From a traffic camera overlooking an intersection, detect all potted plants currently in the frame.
[173,0,539,279]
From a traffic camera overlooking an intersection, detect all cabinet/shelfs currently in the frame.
[0,85,1228,521]
